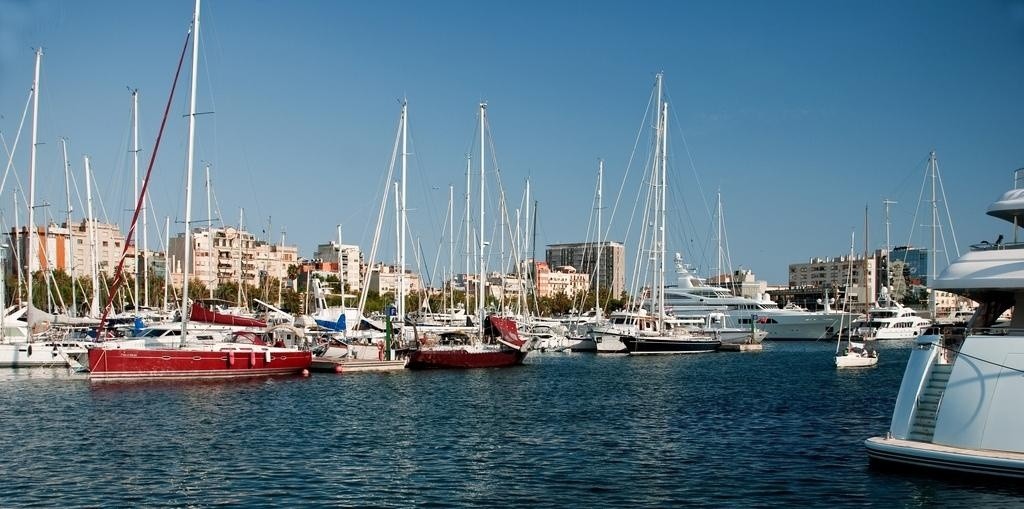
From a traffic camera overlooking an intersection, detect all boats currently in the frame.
[861,166,1023,487]
